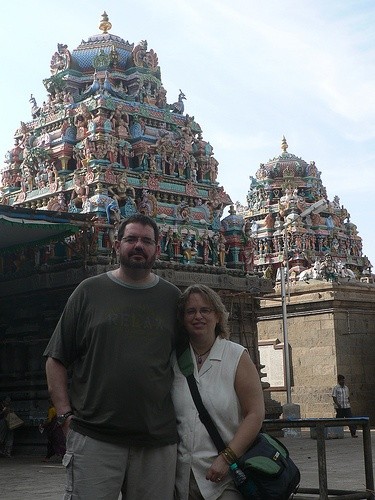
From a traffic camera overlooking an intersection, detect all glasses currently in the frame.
[185,308,217,316]
[120,237,157,246]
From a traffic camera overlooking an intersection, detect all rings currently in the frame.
[217,477,221,481]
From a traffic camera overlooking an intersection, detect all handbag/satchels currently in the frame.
[230,433,300,500]
[4,411,24,430]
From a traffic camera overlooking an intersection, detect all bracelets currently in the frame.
[221,448,238,465]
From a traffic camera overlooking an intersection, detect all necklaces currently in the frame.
[193,348,211,362]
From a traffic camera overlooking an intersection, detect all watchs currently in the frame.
[57,411,72,424]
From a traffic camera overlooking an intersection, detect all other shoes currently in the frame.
[352,435,358,438]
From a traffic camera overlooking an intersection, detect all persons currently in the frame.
[43,215,182,500]
[0,396,16,458]
[237,161,363,284]
[43,400,61,463]
[171,285,265,500]
[0,39,260,270]
[332,375,358,437]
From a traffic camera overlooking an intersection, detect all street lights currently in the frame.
[282,198,329,436]
